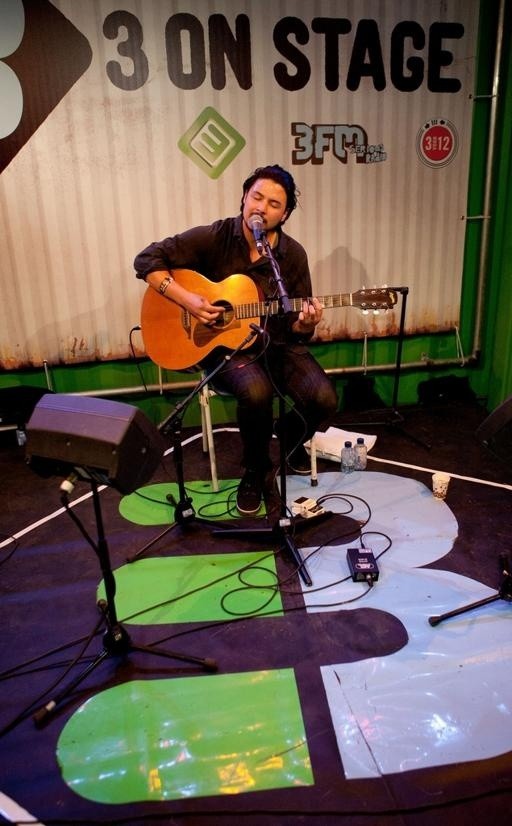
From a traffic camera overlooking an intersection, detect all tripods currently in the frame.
[209,242,335,586]
[429,552,512,627]
[331,293,433,450]
[31,480,218,727]
[125,331,256,564]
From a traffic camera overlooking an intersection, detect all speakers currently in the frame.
[16,390,168,495]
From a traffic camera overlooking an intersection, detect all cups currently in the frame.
[431,473,449,500]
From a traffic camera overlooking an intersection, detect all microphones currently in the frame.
[247,214,265,256]
[249,323,266,334]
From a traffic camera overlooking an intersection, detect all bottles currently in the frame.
[341,438,367,475]
[16,423,26,445]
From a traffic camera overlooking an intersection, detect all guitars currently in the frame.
[140,270,396,374]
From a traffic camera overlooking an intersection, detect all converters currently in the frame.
[346,547,379,582]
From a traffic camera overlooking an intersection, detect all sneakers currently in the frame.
[236,468,262,514]
[285,444,311,473]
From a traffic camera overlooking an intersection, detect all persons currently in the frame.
[132,165,339,519]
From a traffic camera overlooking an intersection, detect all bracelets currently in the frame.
[158,275,175,294]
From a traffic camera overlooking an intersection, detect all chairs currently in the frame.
[198,368,320,495]
